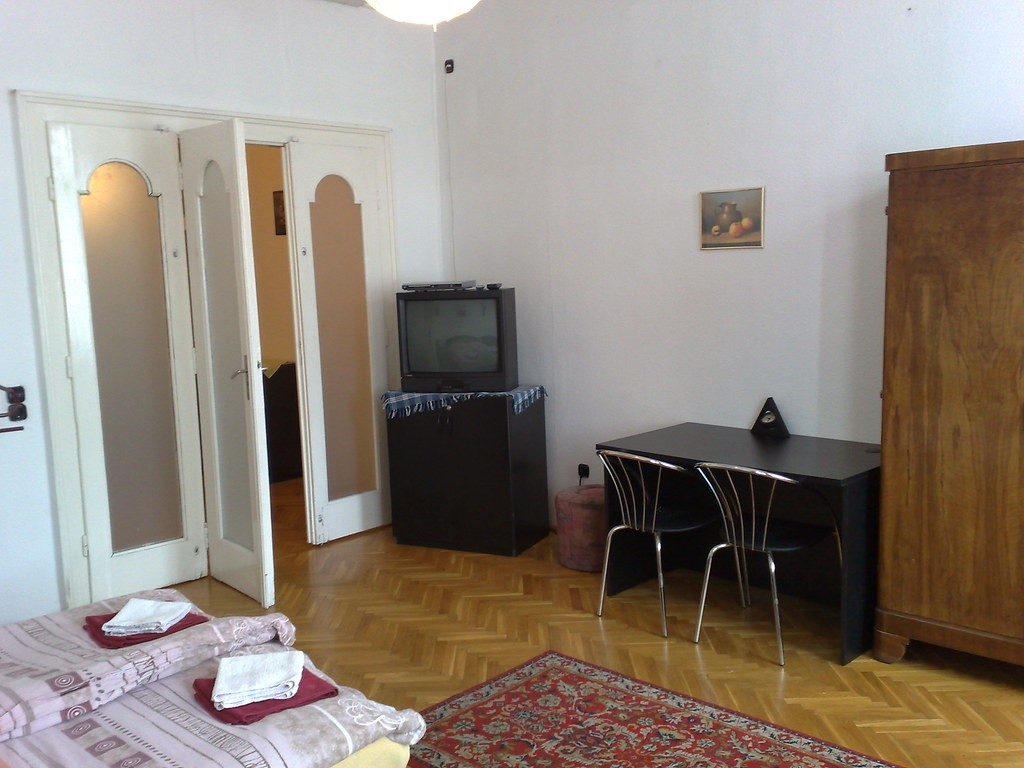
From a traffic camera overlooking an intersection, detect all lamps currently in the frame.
[367,0,482,32]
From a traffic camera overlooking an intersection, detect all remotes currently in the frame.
[487,283,503,289]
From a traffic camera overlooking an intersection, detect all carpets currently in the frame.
[409,649,905,768]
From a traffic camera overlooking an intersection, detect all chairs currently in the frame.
[595,449,746,638]
[693,461,844,668]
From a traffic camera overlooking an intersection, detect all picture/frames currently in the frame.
[273,191,286,235]
[698,186,764,250]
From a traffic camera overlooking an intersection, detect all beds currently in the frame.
[0,588,410,768]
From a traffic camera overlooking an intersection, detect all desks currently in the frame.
[595,423,884,666]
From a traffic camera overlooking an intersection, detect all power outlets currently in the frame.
[578,464,589,478]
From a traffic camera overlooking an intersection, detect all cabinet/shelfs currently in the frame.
[263,362,303,484]
[874,141,1024,664]
[385,392,549,556]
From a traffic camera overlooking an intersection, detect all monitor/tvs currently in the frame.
[396,286,519,393]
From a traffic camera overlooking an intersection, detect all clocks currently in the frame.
[750,398,792,438]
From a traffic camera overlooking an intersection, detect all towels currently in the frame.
[101,598,191,635]
[82,609,209,649]
[212,650,305,712]
[192,665,339,727]
